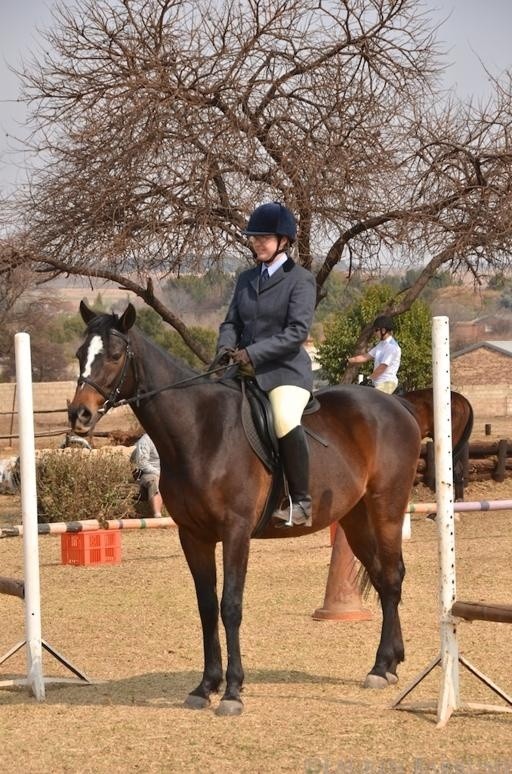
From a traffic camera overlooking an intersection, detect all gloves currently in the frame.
[215,345,250,367]
[359,377,372,386]
[340,359,349,369]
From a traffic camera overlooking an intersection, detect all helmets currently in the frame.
[242,202,297,245]
[374,315,392,329]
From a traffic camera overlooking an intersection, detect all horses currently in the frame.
[66,300,420,718]
[394,389,473,523]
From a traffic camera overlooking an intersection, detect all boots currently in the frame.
[271,425,312,527]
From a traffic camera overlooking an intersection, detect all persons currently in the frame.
[202,200,314,528]
[130,432,164,517]
[340,314,402,395]
